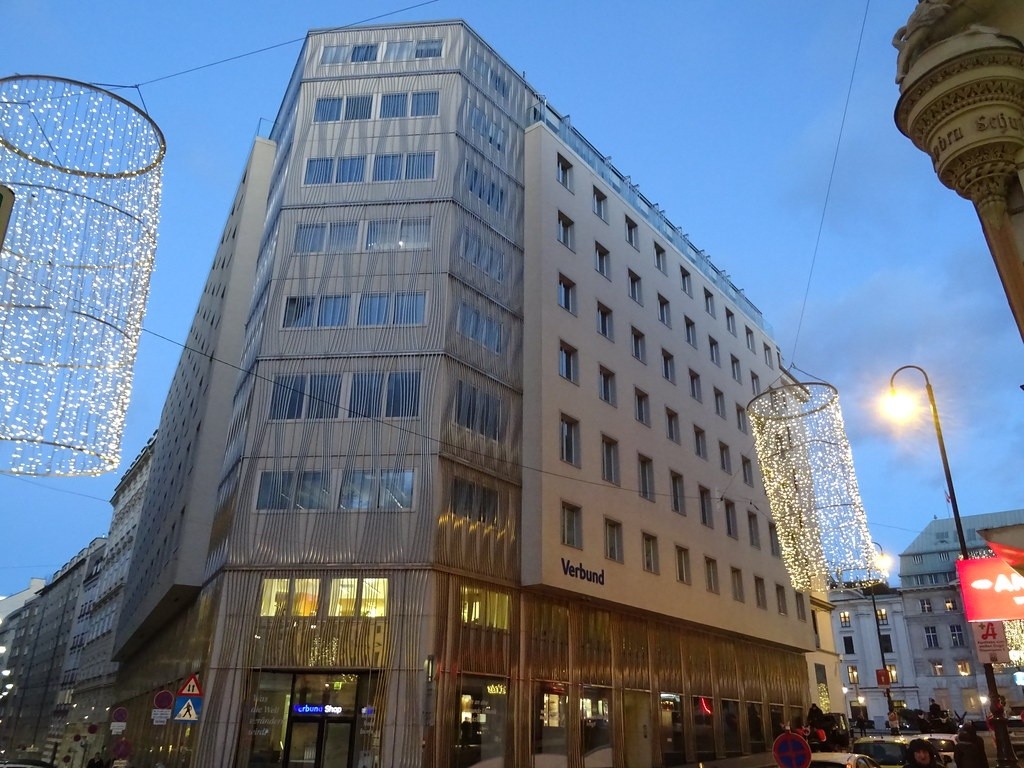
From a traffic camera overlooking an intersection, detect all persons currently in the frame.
[461,717,471,750]
[807,699,989,768]
[87,752,104,768]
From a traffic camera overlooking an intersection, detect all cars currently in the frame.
[809,734,958,768]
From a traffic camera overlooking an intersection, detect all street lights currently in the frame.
[866,541,900,735]
[877,365,1022,768]
